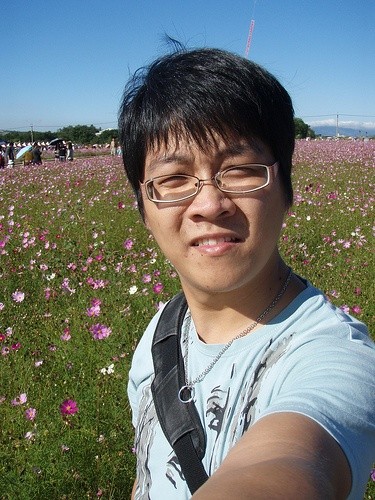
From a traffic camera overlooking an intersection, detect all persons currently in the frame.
[32,142,37,151]
[117,35,375,500]
[109,137,117,155]
[1,144,7,164]
[65,143,73,161]
[6,143,14,165]
[32,144,42,164]
[117,141,122,156]
[0,145,4,167]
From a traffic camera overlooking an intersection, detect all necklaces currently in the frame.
[178,266,295,404]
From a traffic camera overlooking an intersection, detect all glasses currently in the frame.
[136,162,279,203]
